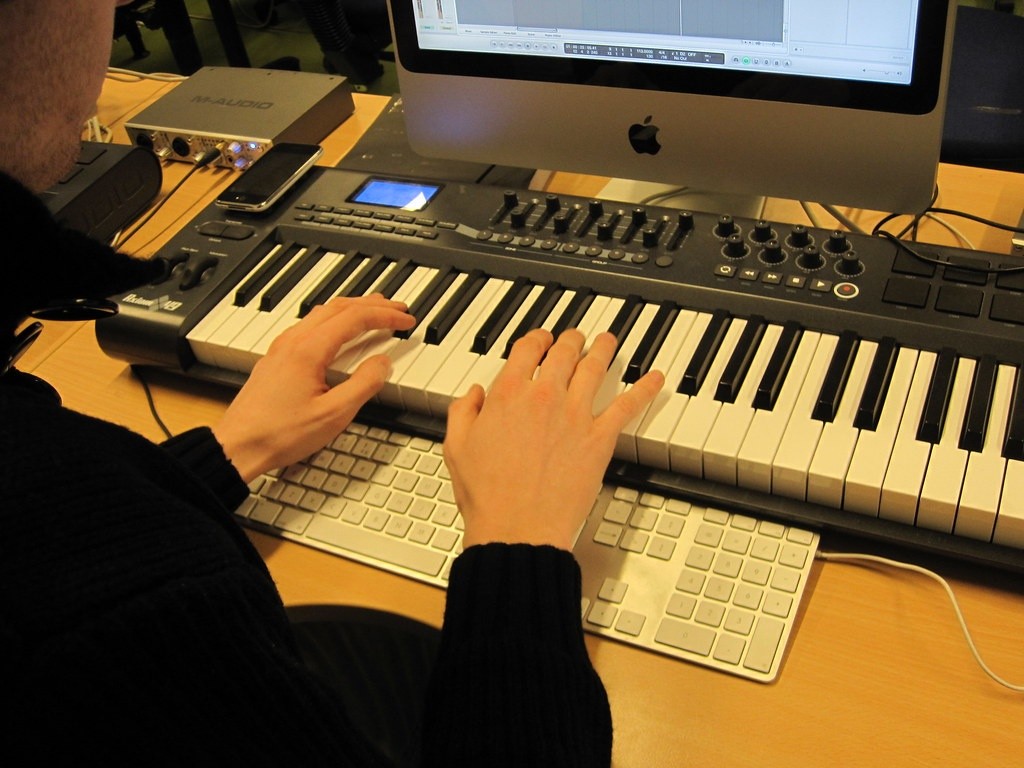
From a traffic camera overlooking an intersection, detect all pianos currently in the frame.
[93,161,1024,601]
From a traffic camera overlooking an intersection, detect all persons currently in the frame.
[2,1,667,768]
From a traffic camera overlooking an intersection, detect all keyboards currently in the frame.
[233,420,821,683]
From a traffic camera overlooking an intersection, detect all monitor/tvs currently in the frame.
[383,0,958,224]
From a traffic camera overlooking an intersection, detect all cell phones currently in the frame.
[214,142,324,211]
